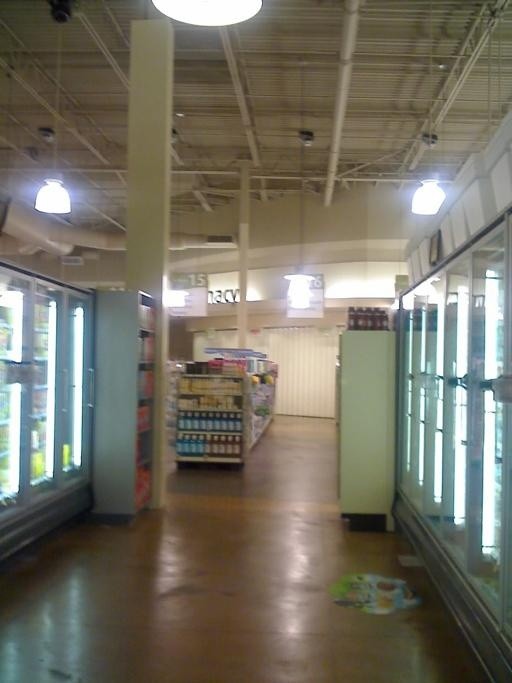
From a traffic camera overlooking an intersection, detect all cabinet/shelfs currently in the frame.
[337,327,397,532]
[178,364,278,471]
[90,287,156,524]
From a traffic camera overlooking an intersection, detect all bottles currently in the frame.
[348,306,389,331]
[175,410,242,458]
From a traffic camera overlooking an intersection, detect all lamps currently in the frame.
[411,1,446,216]
[150,0,262,26]
[34,27,72,215]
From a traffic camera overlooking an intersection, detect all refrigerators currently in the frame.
[390,202,512,683]
[0,259,96,565]
